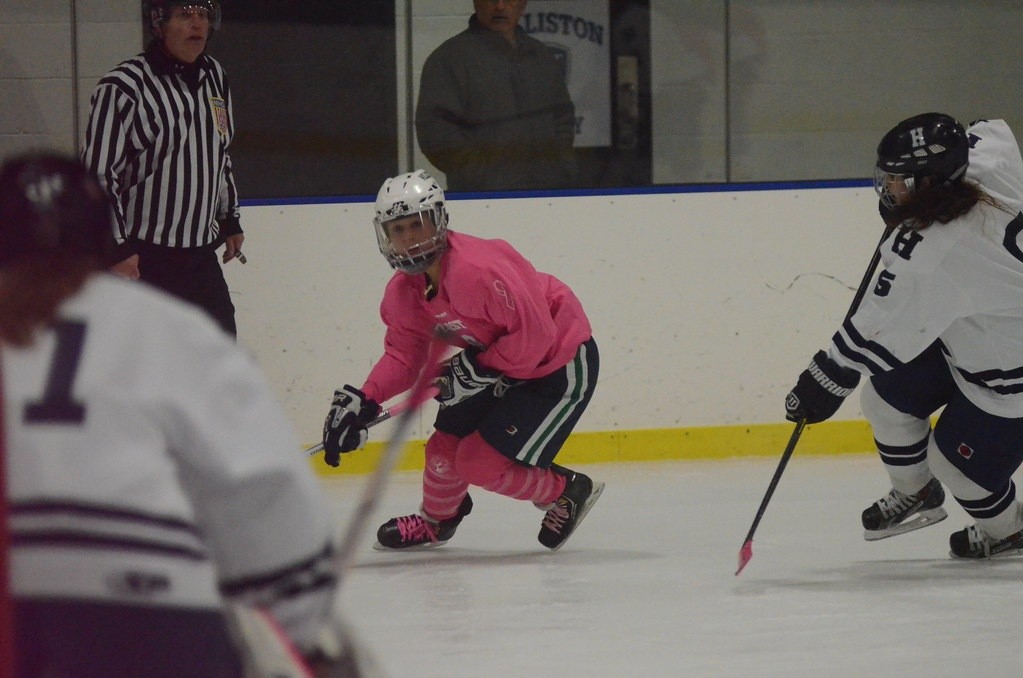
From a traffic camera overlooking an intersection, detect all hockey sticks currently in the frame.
[734,223,896,577]
[303,385,441,458]
[324,340,452,588]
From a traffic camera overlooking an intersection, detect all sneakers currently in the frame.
[374,493,473,554]
[862,473,948,541]
[538,462,604,552]
[949,520,1023,560]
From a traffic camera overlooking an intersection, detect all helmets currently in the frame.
[0,150,106,263]
[373,171,449,275]
[143,0,216,23]
[873,112,969,214]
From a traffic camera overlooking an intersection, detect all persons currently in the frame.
[323,170,606,555]
[786,113,1023,562]
[0,147,364,678]
[415,1,575,191]
[78,0,245,340]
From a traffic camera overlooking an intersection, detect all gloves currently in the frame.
[786,348,861,425]
[431,344,504,407]
[323,384,381,467]
